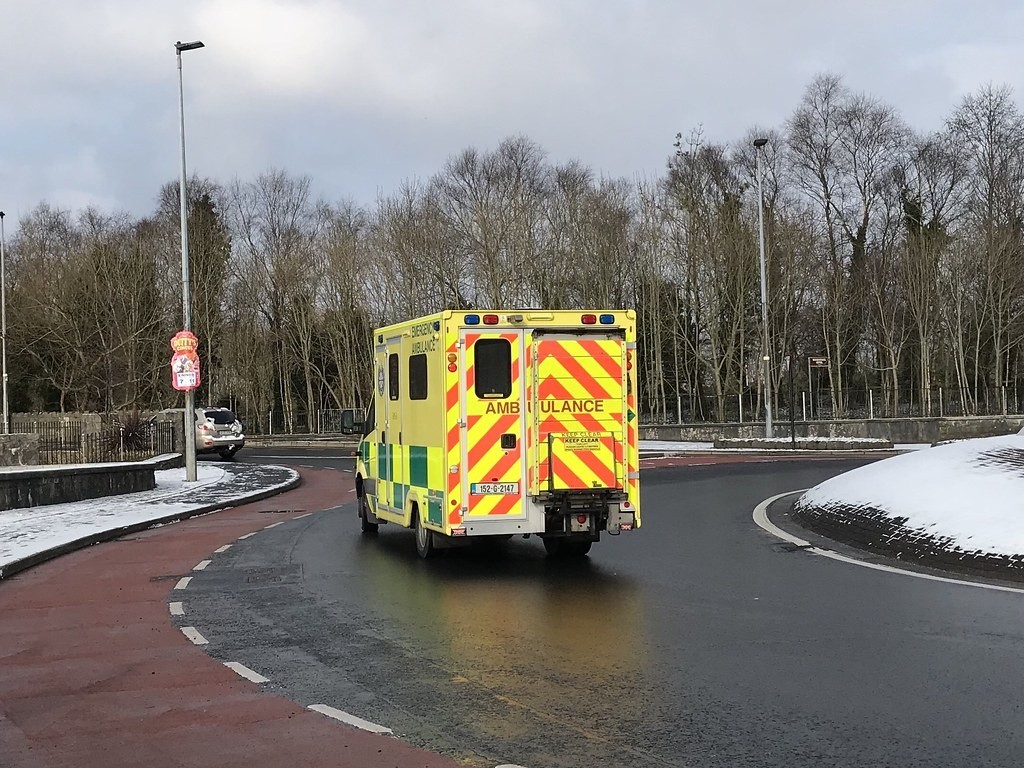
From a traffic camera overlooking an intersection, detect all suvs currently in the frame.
[143,406,245,458]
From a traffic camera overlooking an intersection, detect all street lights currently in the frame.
[0,211,10,434]
[174,41,205,482]
[753,139,773,438]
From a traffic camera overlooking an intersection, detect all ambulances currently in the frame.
[341,309,641,561]
